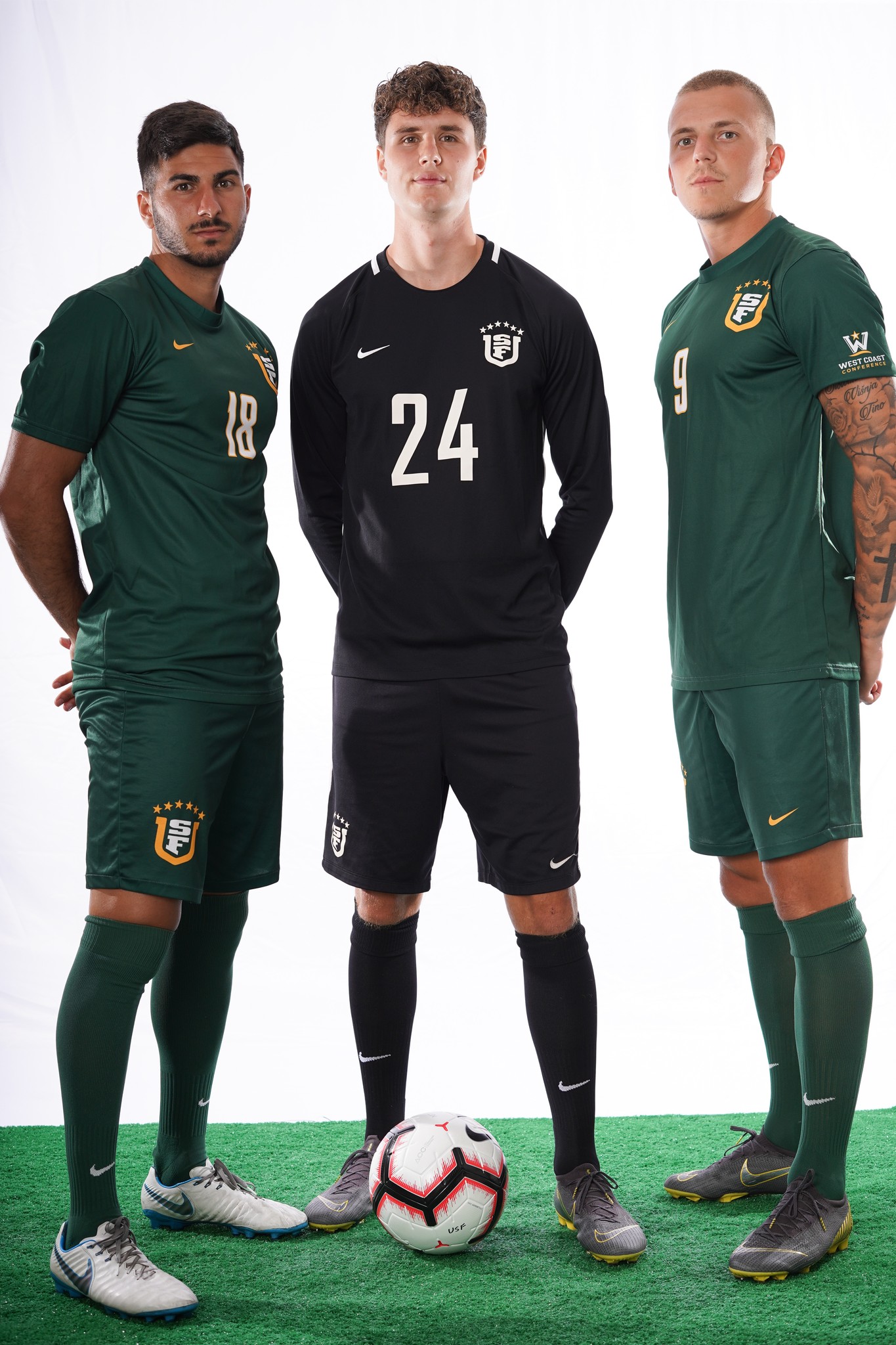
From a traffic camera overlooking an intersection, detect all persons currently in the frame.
[0,100,309,1322]
[650,72,896,1287]
[290,57,647,1264]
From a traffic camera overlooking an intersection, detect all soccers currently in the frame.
[368,1109,511,1257]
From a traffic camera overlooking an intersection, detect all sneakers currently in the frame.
[140,1157,309,1239]
[554,1163,648,1264]
[663,1125,798,1202]
[729,1167,853,1282]
[303,1134,383,1234]
[50,1215,198,1319]
